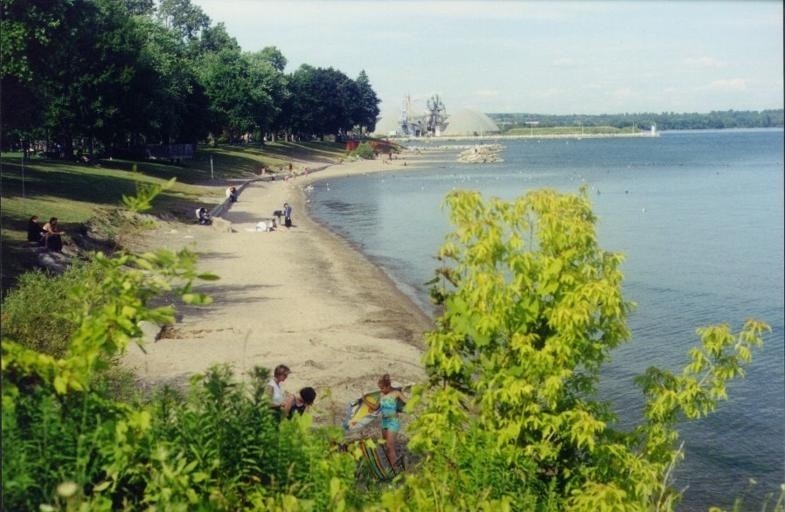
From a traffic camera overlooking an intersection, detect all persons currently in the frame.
[281,202,292,228]
[27,216,49,248]
[283,387,316,421]
[263,364,292,429]
[358,372,409,467]
[40,216,66,254]
[271,209,283,227]
[225,186,238,202]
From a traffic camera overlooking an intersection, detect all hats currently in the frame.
[301,387,316,405]
